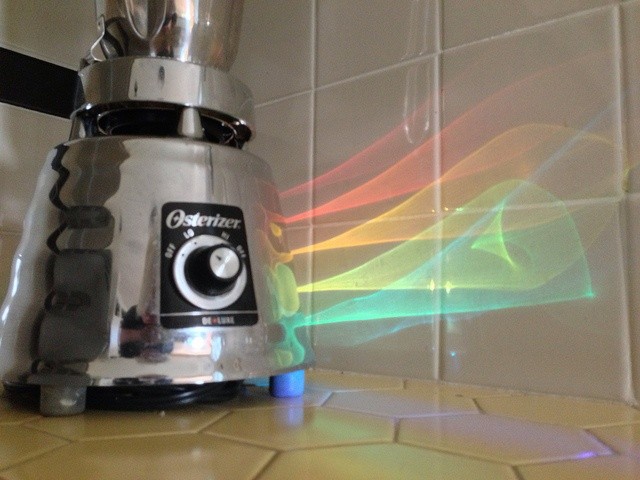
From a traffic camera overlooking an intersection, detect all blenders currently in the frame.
[0,0,316,418]
